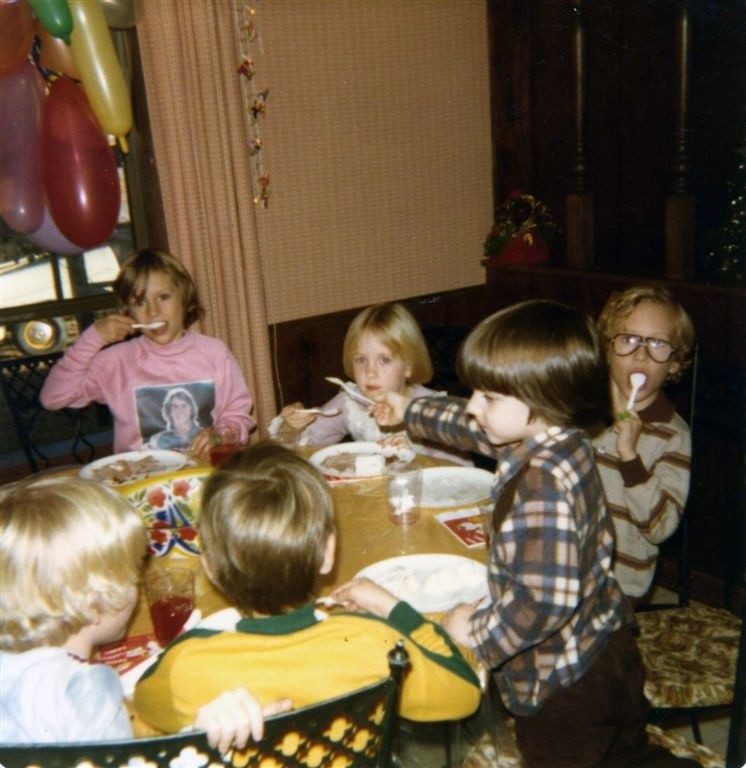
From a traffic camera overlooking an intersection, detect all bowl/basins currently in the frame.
[114,467,216,558]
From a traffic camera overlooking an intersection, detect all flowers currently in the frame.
[478,190,565,254]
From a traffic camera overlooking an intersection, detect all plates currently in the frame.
[389,466,495,507]
[197,606,329,632]
[353,553,492,614]
[309,442,415,477]
[79,450,186,486]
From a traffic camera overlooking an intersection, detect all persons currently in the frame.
[368,298,650,768]
[0,473,293,768]
[148,388,205,447]
[268,302,447,453]
[39,247,260,461]
[132,438,479,768]
[592,279,691,607]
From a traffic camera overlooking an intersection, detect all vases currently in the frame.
[494,234,550,266]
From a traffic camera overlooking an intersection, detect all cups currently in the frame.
[384,464,422,526]
[145,568,194,649]
[207,422,241,468]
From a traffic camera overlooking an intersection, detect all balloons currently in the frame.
[0,0,133,261]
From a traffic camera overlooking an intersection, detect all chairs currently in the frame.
[0,644,409,768]
[635,477,746,768]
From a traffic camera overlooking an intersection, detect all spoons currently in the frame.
[131,322,166,328]
[325,377,376,405]
[298,408,339,415]
[626,373,646,410]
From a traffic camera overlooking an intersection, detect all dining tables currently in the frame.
[0,453,495,768]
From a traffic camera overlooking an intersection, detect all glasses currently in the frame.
[603,332,676,364]
